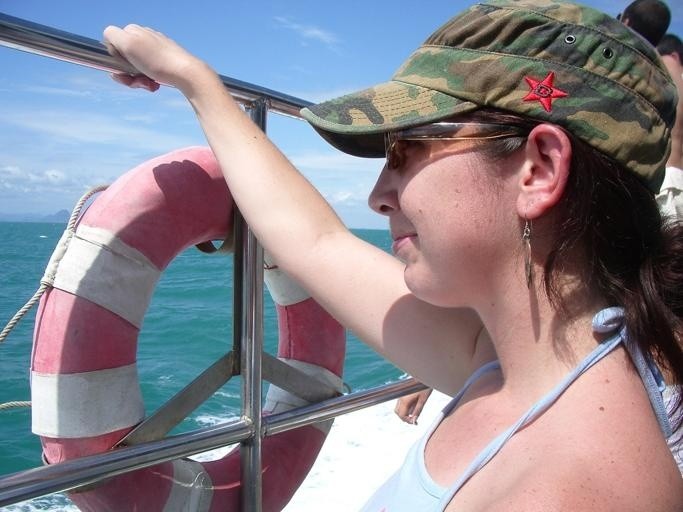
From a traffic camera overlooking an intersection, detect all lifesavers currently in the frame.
[27,144,346,512]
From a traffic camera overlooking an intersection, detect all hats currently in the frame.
[299,2,678,195]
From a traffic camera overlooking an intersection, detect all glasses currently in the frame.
[387,123,518,169]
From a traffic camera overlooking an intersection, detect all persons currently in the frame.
[607,0,673,54]
[626,33,681,235]
[392,307,681,478]
[98,0,682,512]
